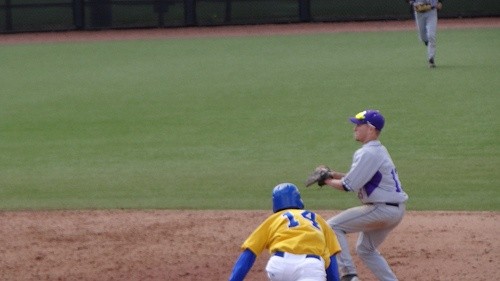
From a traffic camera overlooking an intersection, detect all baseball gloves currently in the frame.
[413,0,432,13]
[303,164,334,187]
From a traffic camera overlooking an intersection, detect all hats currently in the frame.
[349,109,385,130]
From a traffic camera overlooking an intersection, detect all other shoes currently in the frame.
[340,274,359,281]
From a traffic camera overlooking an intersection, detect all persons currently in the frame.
[410,0,443,67]
[227,183,342,281]
[305,109,408,281]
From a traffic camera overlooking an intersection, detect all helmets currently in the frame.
[272,182,304,212]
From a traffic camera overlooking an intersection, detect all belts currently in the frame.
[275,250,321,260]
